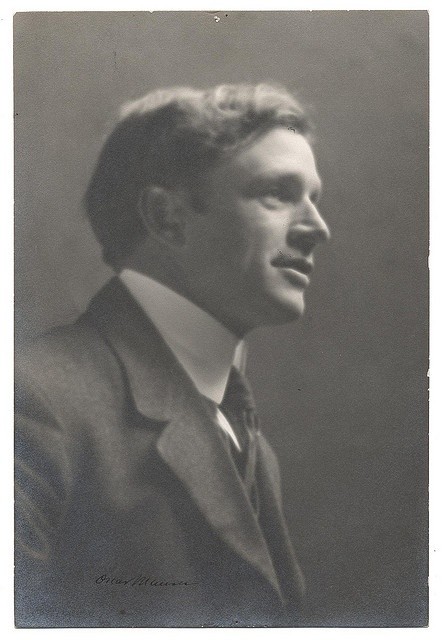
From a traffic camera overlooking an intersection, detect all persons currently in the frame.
[16,81,330,626]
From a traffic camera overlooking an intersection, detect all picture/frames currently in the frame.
[0,0,443,640]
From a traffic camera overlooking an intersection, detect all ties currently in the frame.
[218,366,263,504]
